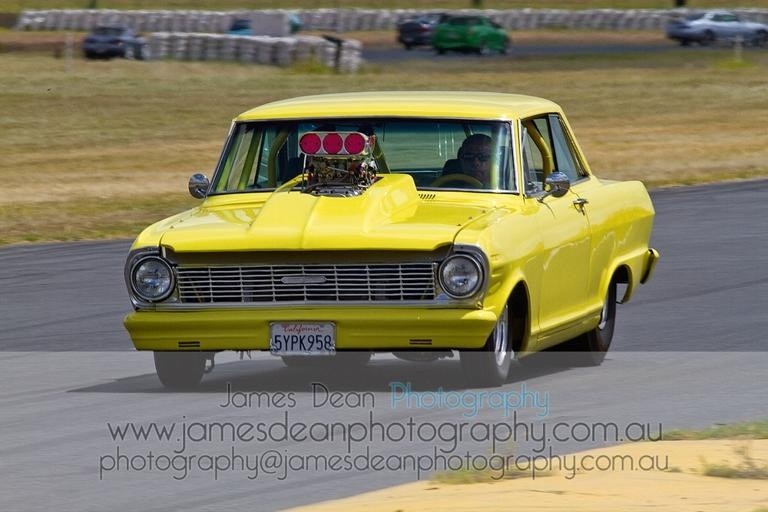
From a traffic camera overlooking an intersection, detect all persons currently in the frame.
[463,134,491,189]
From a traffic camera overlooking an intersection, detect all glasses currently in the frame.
[461,150,490,161]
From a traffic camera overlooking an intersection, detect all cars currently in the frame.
[667,11,767,48]
[224,14,299,39]
[433,16,509,56]
[84,23,147,61]
[399,14,445,46]
[122,88,659,385]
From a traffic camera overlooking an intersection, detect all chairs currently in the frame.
[442,160,466,176]
[280,158,299,183]
